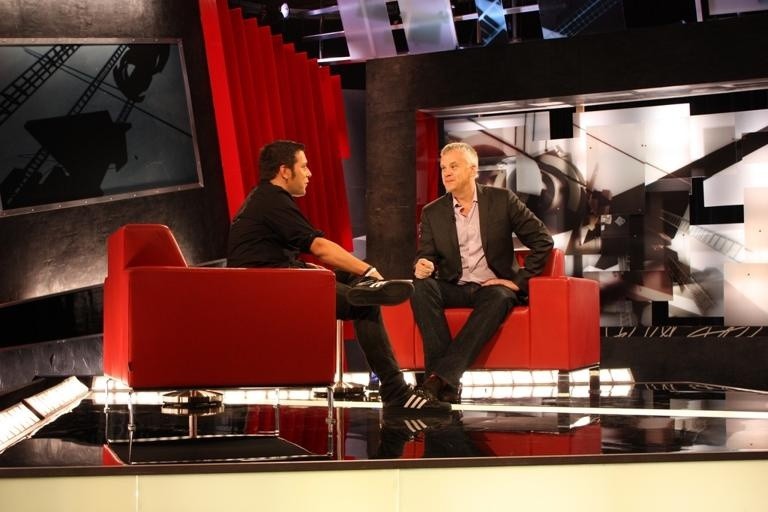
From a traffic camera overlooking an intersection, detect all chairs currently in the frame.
[104,224,338,466]
[380,251,600,431]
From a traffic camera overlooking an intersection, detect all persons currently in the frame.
[349,411,452,459]
[423,410,484,460]
[227,140,454,415]
[410,143,555,403]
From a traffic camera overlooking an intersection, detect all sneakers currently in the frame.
[383,374,462,416]
[382,415,453,442]
[346,279,415,306]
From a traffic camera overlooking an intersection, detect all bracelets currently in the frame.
[363,264,376,279]
